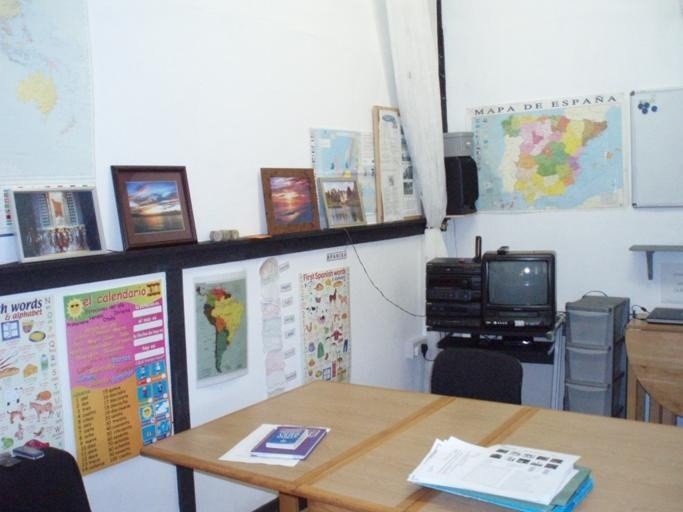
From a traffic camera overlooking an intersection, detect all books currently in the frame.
[405,435,593,512]
[265,428,308,451]
[251,422,326,460]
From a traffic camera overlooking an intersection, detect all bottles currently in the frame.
[40,354,48,375]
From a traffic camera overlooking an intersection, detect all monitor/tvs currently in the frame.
[482,251,556,328]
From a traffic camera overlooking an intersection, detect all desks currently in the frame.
[140,379,683,512]
[624,312,682,426]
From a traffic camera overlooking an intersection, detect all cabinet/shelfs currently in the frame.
[564,296,630,419]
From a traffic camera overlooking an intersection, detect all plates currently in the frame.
[28,331,46,343]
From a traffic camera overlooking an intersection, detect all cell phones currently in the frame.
[13,445,45,460]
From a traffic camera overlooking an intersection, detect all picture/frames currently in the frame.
[261,168,321,236]
[111,166,198,252]
[319,177,367,230]
[7,182,110,264]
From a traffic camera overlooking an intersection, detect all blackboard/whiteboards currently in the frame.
[629,87,683,208]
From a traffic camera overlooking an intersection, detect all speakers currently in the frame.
[445,156,479,214]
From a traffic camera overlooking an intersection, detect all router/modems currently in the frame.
[426,236,483,274]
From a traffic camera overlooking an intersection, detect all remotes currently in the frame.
[497,245,509,254]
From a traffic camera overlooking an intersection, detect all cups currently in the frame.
[22,320,33,334]
[209,230,239,243]
